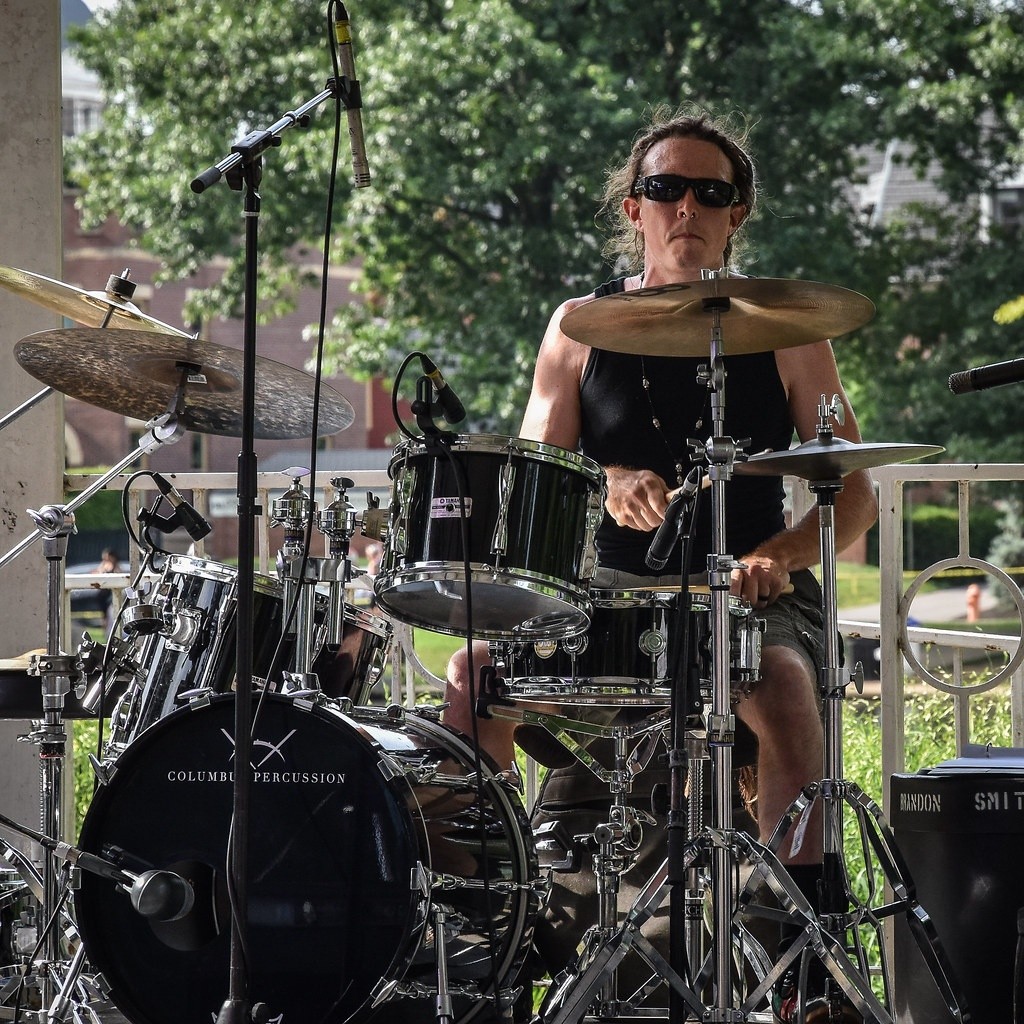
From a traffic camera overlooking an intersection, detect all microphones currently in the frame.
[1,811,196,924]
[334,0,372,188]
[419,354,466,426]
[151,471,213,541]
[948,357,1024,396]
[643,466,702,572]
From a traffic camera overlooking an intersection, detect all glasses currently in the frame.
[632,174,738,207]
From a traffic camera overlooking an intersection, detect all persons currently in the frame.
[440,114,885,967]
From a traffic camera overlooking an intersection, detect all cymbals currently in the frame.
[560,276,878,358]
[0,263,192,337]
[729,434,945,481]
[12,325,355,442]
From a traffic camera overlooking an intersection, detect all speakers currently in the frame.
[888,768,1024,1023]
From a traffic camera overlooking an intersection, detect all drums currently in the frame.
[375,430,606,642]
[106,555,394,753]
[490,591,767,705]
[68,685,550,1024]
